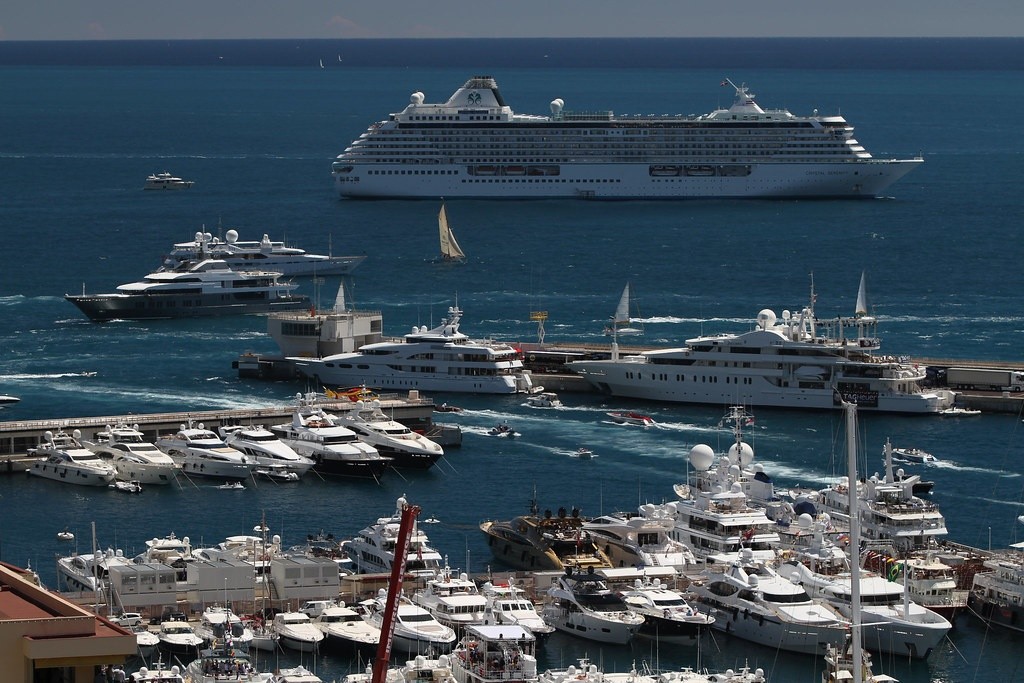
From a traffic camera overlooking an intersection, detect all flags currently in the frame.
[888,563,902,582]
[720,79,729,86]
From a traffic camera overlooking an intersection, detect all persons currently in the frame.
[202,659,252,678]
[850,354,904,363]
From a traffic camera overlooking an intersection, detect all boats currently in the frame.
[331,75,925,203]
[54,406,1024,682]
[63,254,313,324]
[488,423,522,438]
[606,412,656,427]
[688,166,714,175]
[887,446,940,463]
[284,306,545,396]
[433,403,461,412]
[939,405,983,416]
[562,270,956,416]
[156,217,368,279]
[27,394,445,488]
[476,165,497,175]
[217,482,245,489]
[718,166,749,176]
[143,171,194,190]
[502,166,526,175]
[528,392,563,409]
[578,447,593,460]
[650,167,678,175]
[530,166,559,175]
[115,481,143,496]
